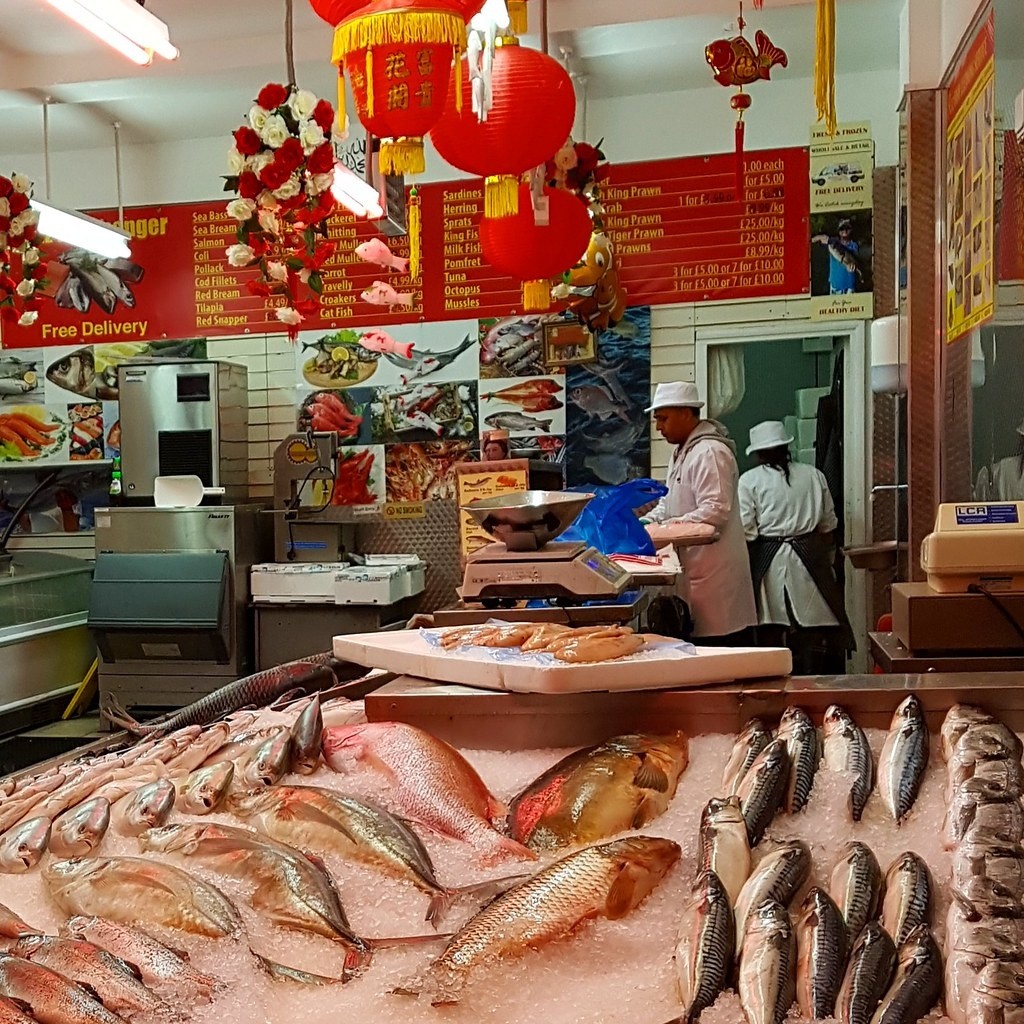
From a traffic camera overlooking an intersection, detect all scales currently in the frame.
[462,542,633,607]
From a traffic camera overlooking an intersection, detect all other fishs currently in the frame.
[2,622,1024,1024]
[826,236,867,284]
[299,314,644,509]
[354,237,416,360]
[558,232,628,333]
[705,30,788,88]
[52,247,144,315]
[1,338,200,462]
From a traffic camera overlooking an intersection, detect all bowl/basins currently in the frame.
[459,490,596,542]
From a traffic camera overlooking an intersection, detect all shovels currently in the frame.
[154,475,224,507]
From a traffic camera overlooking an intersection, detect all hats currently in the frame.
[642,382,705,411]
[745,421,795,456]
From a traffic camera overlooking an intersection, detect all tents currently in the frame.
[309,0,593,312]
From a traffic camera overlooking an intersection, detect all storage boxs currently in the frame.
[1,548,98,737]
[334,565,407,605]
[250,561,350,603]
[364,553,427,598]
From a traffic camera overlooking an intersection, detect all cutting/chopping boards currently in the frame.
[303,354,378,387]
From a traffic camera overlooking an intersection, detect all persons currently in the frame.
[627,379,759,647]
[481,436,509,459]
[971,421,1024,503]
[737,420,856,674]
[812,218,862,294]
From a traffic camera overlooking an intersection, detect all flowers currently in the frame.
[220,83,351,343]
[546,134,614,218]
[0,172,48,327]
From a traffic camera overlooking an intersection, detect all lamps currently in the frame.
[451,0,511,66]
[28,100,133,260]
[46,0,180,67]
[329,157,384,219]
